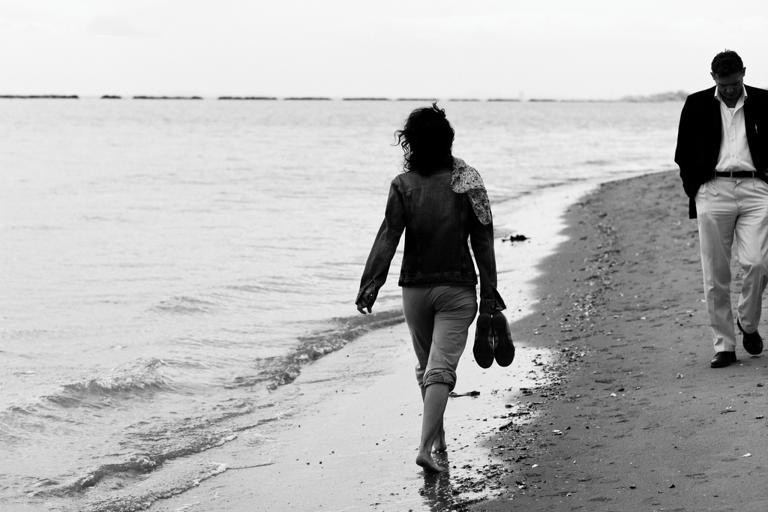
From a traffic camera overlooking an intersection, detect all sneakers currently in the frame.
[491,313,514,366]
[472,314,494,369]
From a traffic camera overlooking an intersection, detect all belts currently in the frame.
[715,171,757,178]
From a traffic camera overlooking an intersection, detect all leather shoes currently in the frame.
[736,319,762,355]
[710,351,736,368]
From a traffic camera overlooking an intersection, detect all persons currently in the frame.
[354,100,508,474]
[672,49,768,370]
